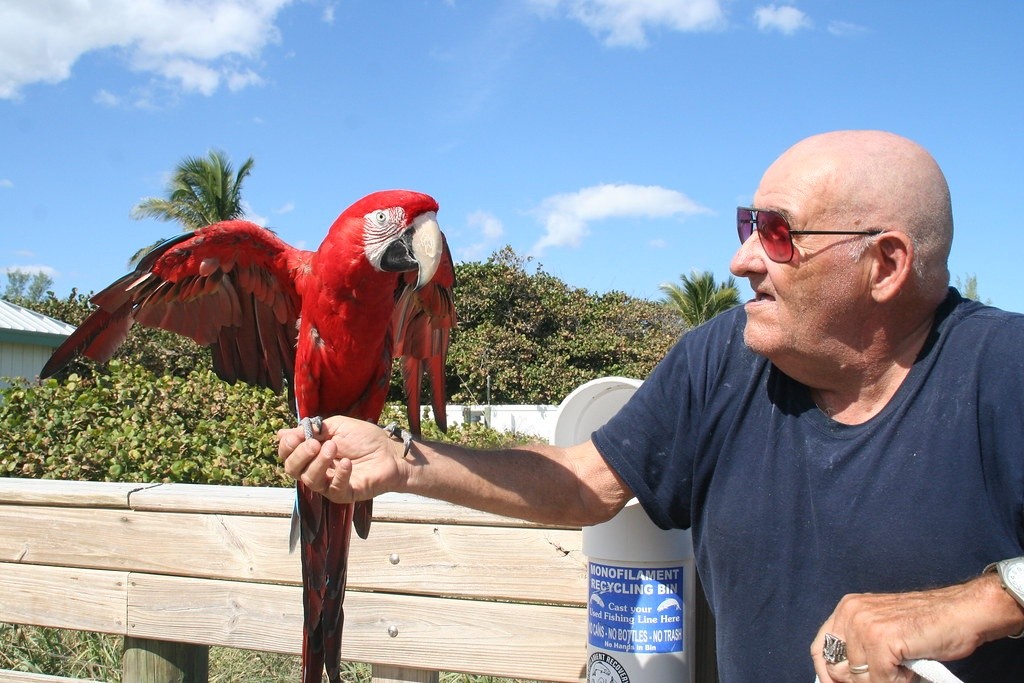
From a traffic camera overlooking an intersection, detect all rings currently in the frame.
[848,664,869,674]
[823,633,847,664]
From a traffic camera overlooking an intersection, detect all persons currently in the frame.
[277,127,1024,683]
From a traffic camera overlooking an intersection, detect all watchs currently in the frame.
[984,556,1024,640]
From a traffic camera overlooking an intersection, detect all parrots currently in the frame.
[40,189,461,682]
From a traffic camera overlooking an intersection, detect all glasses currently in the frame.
[737,206,881,263]
